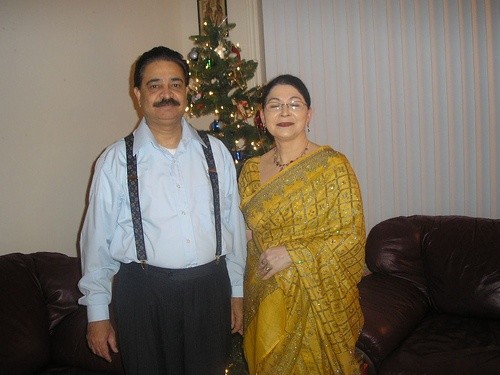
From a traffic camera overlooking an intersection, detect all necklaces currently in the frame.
[273,141,310,173]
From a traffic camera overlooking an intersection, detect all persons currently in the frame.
[78,45,247,375]
[237,73,367,375]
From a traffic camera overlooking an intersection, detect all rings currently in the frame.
[265,266,270,271]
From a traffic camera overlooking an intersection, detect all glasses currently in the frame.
[265,100,307,110]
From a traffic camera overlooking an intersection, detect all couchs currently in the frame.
[0,252,124,375]
[356,215,500,375]
